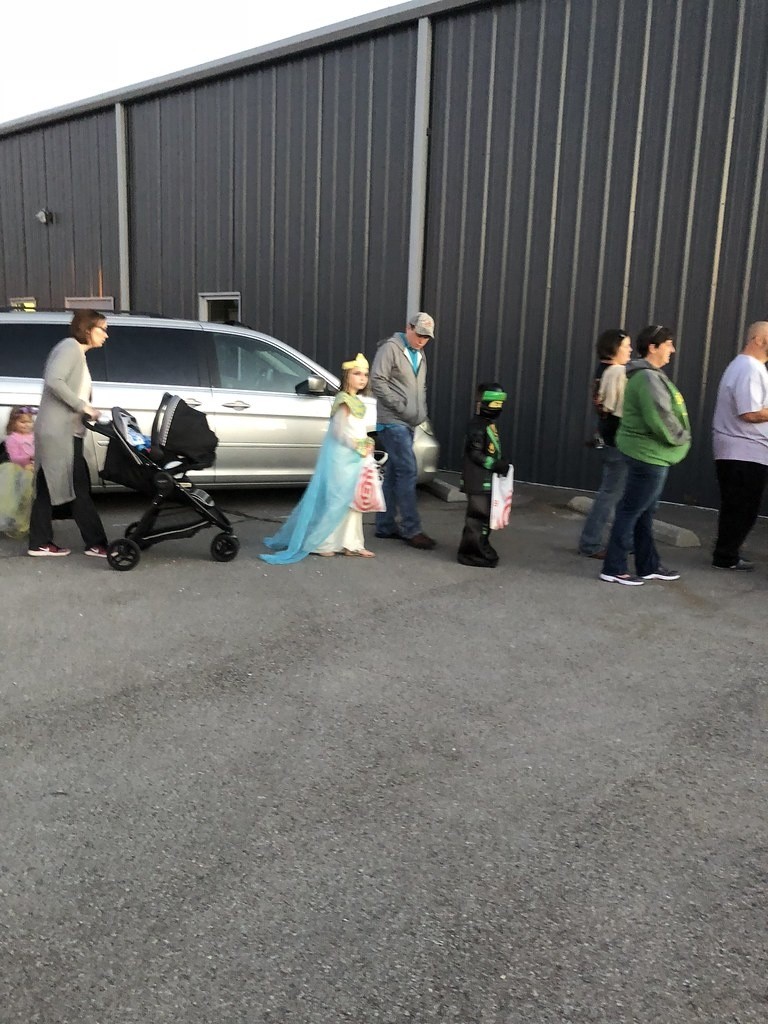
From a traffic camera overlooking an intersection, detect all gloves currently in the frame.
[492,461,510,477]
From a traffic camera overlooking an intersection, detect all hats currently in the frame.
[409,312,435,339]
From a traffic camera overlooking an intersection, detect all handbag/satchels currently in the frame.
[489,464,514,530]
[349,450,389,512]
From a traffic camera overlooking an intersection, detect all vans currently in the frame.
[0,311,438,520]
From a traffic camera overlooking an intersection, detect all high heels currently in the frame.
[318,552,336,556]
[344,548,375,558]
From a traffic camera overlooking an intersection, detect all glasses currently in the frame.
[94,324,108,334]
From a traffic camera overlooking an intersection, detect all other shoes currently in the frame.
[375,531,400,539]
[401,534,435,548]
[712,557,754,572]
[588,550,607,559]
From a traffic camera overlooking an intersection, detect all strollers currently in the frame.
[83,393,241,570]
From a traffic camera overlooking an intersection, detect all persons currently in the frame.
[259,353,375,564]
[458,384,510,565]
[579,329,633,556]
[371,312,438,550]
[27,308,120,557]
[712,321,768,571]
[0,406,38,536]
[599,326,692,584]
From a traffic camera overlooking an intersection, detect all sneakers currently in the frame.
[27,541,71,556]
[599,569,644,586]
[637,564,680,580]
[84,544,119,557]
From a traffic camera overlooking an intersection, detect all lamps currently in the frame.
[36,207,56,225]
